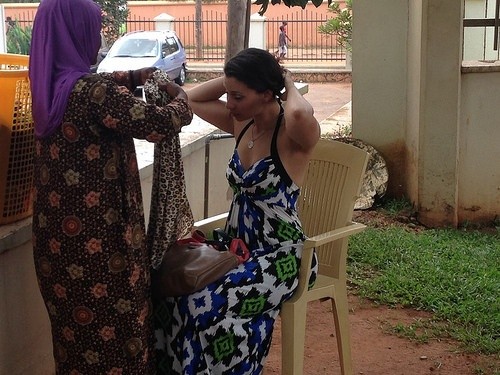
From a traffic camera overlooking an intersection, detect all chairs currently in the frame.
[192,139,370,375]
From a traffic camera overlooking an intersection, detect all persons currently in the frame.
[277,21,291,63]
[28,1,189,375]
[117,20,127,38]
[153,48,320,375]
[6,17,14,34]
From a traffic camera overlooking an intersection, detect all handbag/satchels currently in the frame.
[158,228,249,298]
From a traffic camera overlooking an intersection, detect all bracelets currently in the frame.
[128,70,138,90]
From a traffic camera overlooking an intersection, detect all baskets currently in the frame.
[0,54,36,225]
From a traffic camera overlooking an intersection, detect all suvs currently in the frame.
[96,29,187,86]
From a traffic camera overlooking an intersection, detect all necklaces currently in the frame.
[247,122,269,148]
